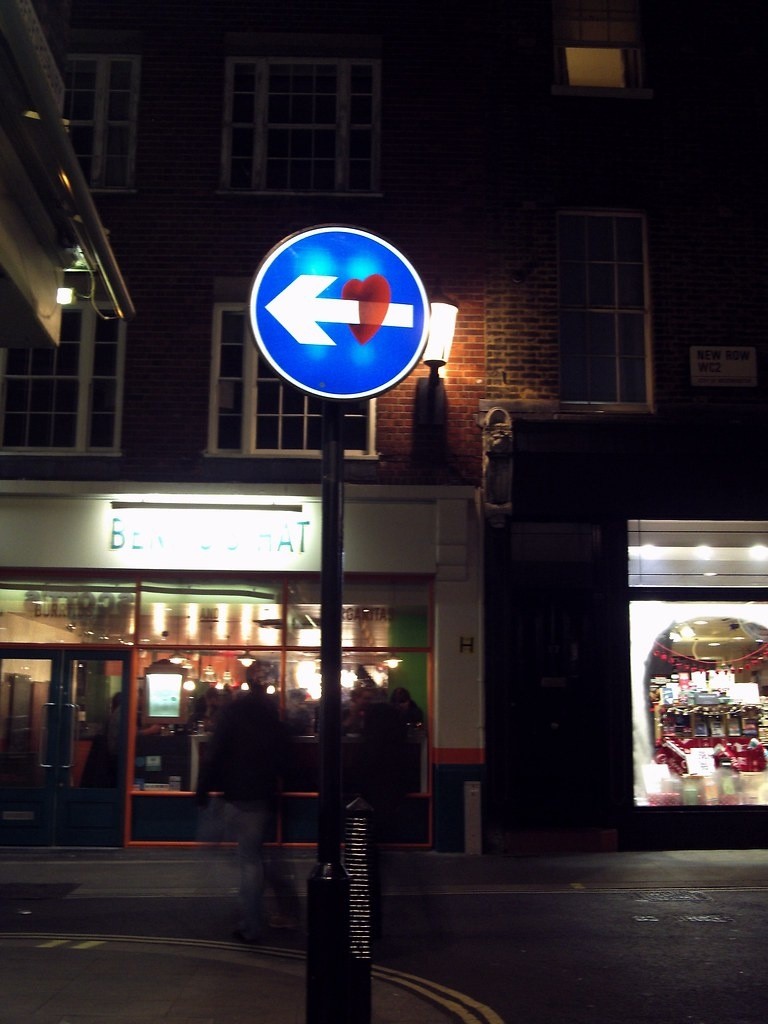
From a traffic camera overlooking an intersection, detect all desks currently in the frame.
[657,737,765,775]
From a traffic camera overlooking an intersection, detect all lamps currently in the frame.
[57,287,91,305]
[223,635,231,680]
[237,650,257,667]
[204,625,214,675]
[167,615,187,664]
[413,283,460,458]
[382,653,403,668]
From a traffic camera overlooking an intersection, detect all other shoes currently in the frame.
[231,925,267,945]
[268,915,298,928]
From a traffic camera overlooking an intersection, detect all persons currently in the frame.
[106,660,425,941]
[747,738,768,763]
